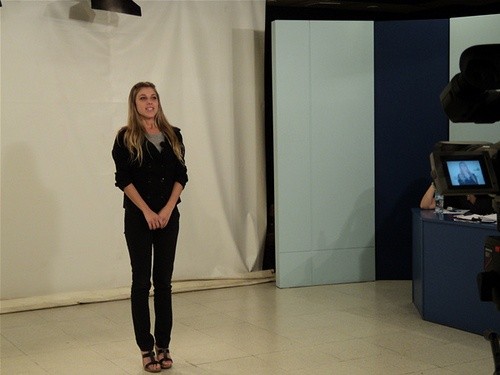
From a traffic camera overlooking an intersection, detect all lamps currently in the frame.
[91,0,141,16]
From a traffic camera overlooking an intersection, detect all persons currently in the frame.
[420,182,496,215]
[458,162,478,185]
[112,82,189,371]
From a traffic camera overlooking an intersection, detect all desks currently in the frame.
[412,207,500,336]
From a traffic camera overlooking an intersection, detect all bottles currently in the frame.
[433,191,444,221]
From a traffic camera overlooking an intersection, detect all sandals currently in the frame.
[143,351,161,373]
[155,347,173,369]
